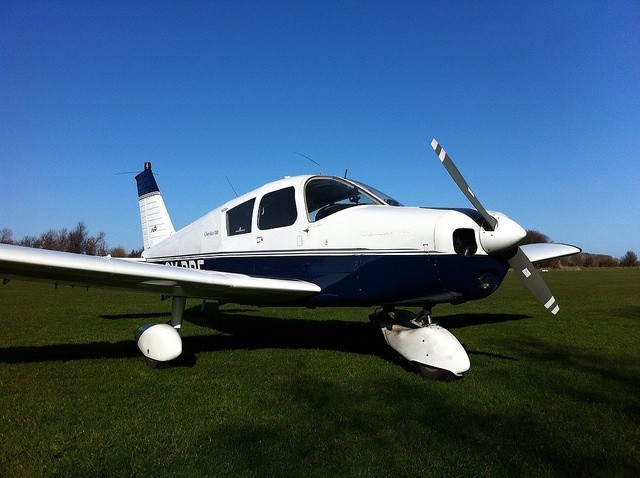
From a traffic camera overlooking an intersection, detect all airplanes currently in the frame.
[0,138,582,377]
